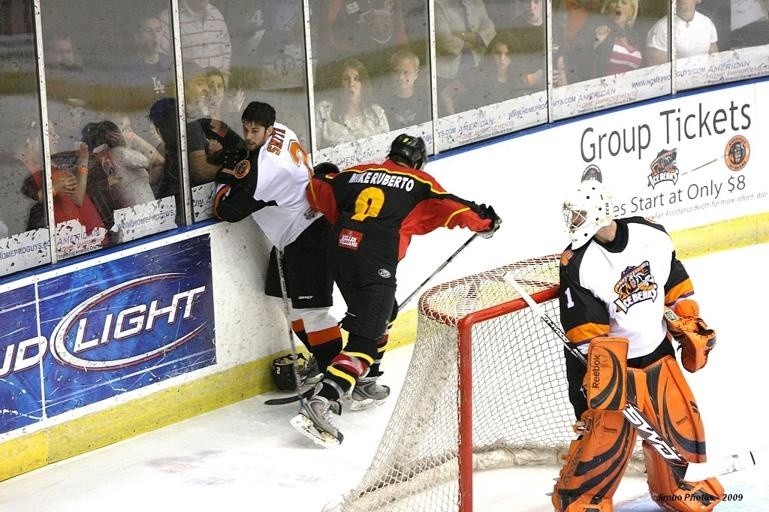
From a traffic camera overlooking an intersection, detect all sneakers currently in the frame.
[301,353,391,441]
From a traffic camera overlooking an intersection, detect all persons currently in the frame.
[551,178,724,512]
[213,101,352,411]
[304,131,498,443]
[1,1,722,247]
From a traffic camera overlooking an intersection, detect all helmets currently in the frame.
[390,134,427,170]
[274,356,307,392]
[562,178,614,250]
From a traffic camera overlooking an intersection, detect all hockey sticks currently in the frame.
[272,239,341,414]
[264,217,502,405]
[504,269,755,484]
